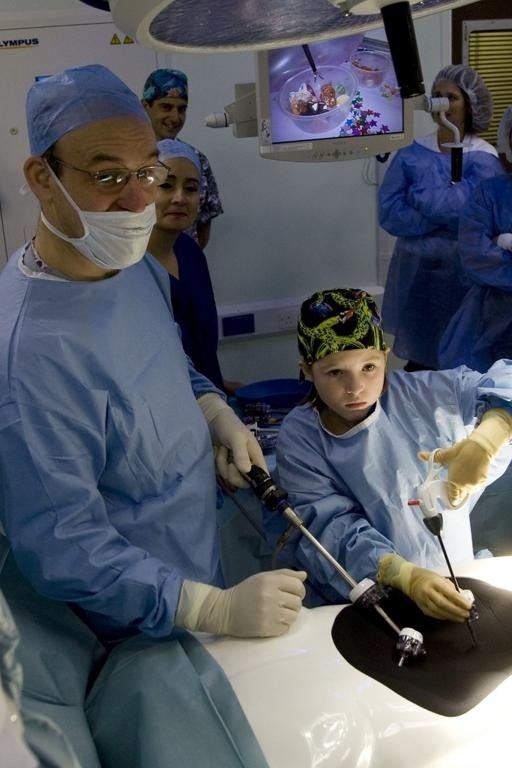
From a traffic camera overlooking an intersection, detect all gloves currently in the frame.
[195,390,273,490]
[415,408,512,511]
[174,567,308,639]
[377,551,474,635]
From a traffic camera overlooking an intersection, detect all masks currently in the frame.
[18,157,158,270]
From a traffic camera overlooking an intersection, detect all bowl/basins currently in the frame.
[278,65,359,134]
[349,51,393,89]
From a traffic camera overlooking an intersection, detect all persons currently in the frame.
[1,60,309,650]
[143,139,247,401]
[261,284,512,624]
[373,62,505,373]
[137,63,225,256]
[429,104,510,376]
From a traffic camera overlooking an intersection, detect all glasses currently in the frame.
[52,159,171,194]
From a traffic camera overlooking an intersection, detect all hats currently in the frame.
[24,63,152,158]
[156,138,202,180]
[496,106,512,164]
[298,286,387,367]
[430,64,493,133]
[142,68,189,104]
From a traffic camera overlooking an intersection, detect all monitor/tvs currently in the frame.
[254,24,413,162]
